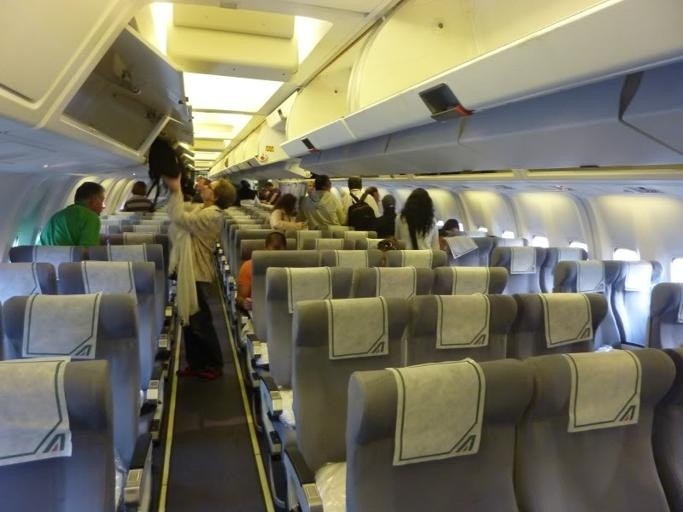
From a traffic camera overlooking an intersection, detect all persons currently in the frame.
[163,174,237,385]
[236,232,287,310]
[269,175,459,250]
[36,181,104,246]
[233,180,281,208]
[122,181,156,212]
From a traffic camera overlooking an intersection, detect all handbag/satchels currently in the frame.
[149,137,181,179]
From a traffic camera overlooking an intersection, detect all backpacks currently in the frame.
[348,192,376,231]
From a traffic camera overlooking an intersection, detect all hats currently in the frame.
[382,195,395,209]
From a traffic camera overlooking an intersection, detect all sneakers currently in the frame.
[193,370,222,381]
[176,367,196,377]
